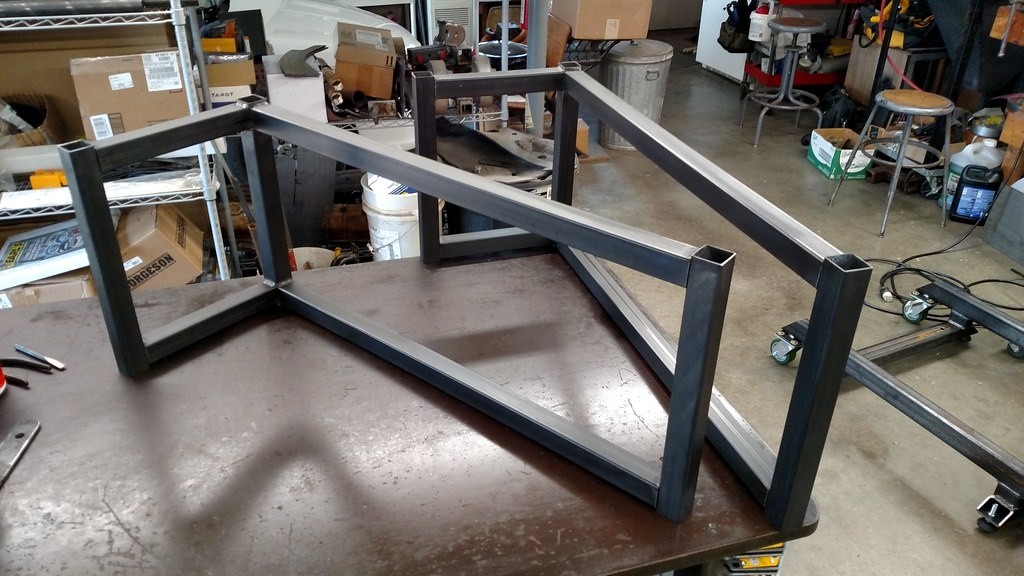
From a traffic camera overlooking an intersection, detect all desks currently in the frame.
[0,252,819,576]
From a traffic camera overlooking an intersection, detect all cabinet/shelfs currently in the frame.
[745,0,865,87]
[0,0,508,310]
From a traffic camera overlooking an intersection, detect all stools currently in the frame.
[828,89,956,238]
[738,17,826,148]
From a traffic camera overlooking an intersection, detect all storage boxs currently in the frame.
[807,114,967,180]
[1,1,652,308]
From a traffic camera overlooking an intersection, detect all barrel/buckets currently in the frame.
[949,164,1004,227]
[599,38,674,151]
[937,139,1003,210]
[747,0,779,42]
[256,247,346,276]
[360,171,445,262]
[470,39,528,71]
